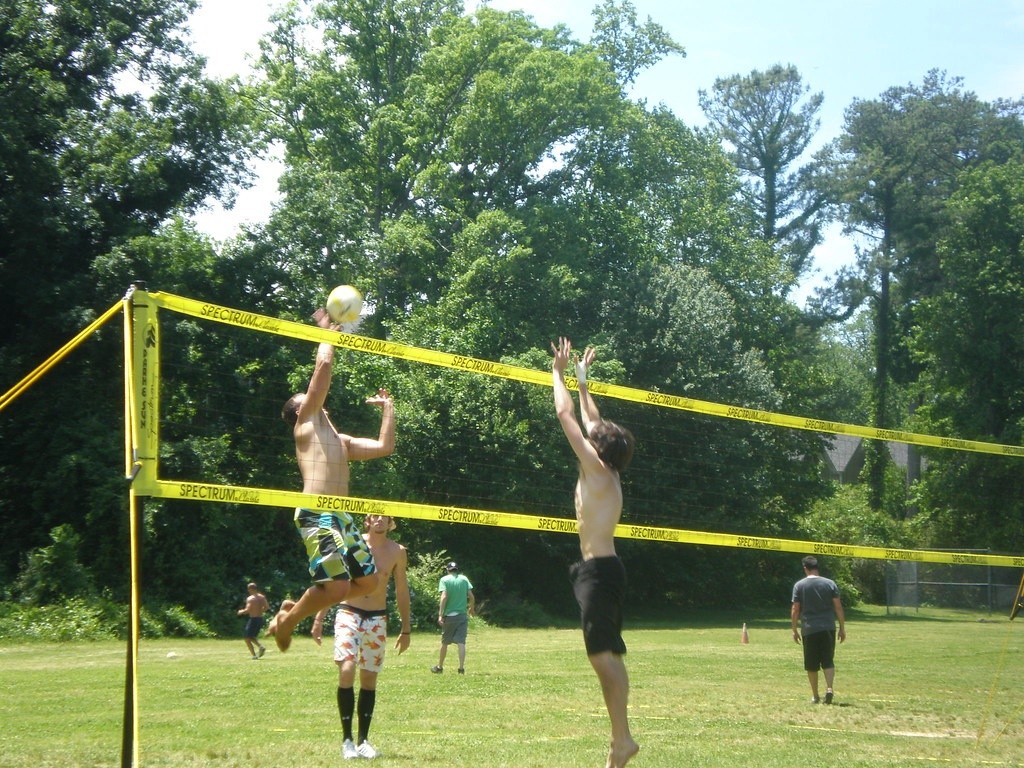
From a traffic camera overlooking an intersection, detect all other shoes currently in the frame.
[431,666,442,673]
[259,646,266,657]
[458,668,465,674]
[823,689,833,704]
[812,697,820,703]
[249,656,258,660]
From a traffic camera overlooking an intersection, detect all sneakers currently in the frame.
[341,739,357,759]
[355,740,378,758]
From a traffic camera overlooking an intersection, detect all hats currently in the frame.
[446,562,458,571]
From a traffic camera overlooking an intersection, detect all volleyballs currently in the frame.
[326,284,364,324]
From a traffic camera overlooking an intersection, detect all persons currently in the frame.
[312,504,414,760]
[792,554,847,704]
[237,582,272,660]
[429,562,475,676]
[264,306,398,652]
[550,335,639,767]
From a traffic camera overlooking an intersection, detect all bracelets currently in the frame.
[401,631,412,635]
